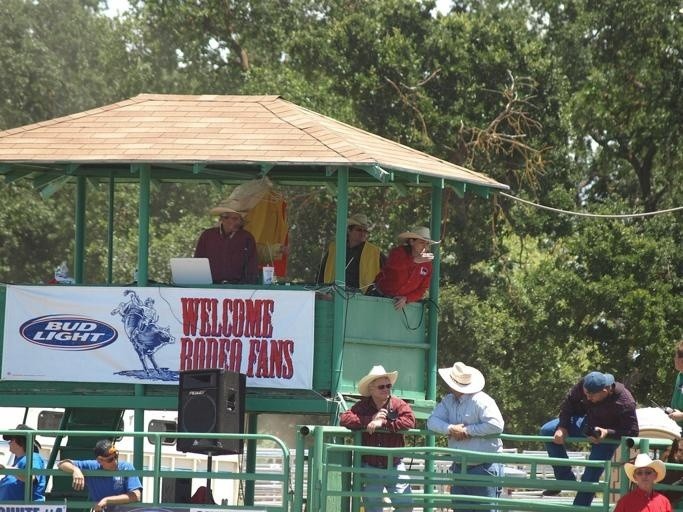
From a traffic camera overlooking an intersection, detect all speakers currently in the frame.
[177,368,246,455]
[162,478,192,503]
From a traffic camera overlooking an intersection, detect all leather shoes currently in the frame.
[543,490,561,497]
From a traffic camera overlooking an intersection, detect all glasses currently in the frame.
[633,468,655,476]
[671,350,682,358]
[368,384,392,390]
[99,450,119,462]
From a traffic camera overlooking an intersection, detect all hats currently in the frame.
[398,226,441,244]
[583,372,615,395]
[438,362,485,394]
[358,366,398,397]
[624,454,666,483]
[209,200,248,219]
[3,424,41,453]
[187,487,215,506]
[94,440,119,455]
[346,214,376,232]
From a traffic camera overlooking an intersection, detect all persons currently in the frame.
[319,213,386,295]
[428,360,505,512]
[609,452,673,512]
[668,340,683,430]
[365,225,441,310]
[194,199,257,284]
[1,424,48,503]
[340,366,417,511]
[539,371,640,512]
[57,441,143,512]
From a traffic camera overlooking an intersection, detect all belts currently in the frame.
[366,463,398,470]
[467,464,482,469]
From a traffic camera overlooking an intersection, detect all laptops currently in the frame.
[170,257,213,286]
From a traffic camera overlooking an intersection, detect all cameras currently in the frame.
[386,410,398,421]
[568,414,601,439]
